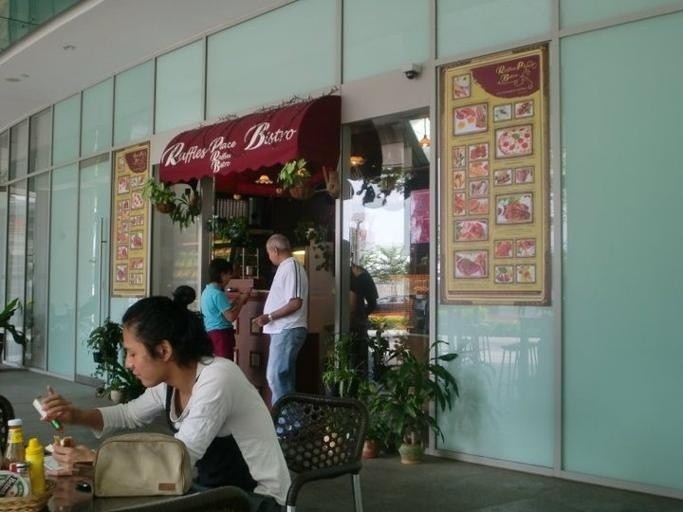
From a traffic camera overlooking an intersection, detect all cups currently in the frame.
[245,266,255,276]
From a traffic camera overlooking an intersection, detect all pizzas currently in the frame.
[132,192,143,208]
[498,124,532,157]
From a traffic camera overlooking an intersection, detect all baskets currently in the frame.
[0,479,56,512]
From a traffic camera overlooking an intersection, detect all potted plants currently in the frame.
[278,158,316,201]
[141,177,177,214]
[86,317,134,402]
[321,333,390,458]
[359,339,459,464]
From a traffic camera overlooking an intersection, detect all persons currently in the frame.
[255,233,309,440]
[200,258,249,363]
[40,286,296,512]
[341,241,379,399]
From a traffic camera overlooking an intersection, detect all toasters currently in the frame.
[3,418,25,467]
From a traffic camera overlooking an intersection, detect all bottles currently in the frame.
[25,437,45,497]
[31,398,64,432]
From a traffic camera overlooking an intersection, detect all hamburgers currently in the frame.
[496,170,511,184]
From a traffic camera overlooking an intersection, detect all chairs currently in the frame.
[269,391,368,511]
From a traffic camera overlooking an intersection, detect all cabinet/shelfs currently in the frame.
[238,247,260,280]
